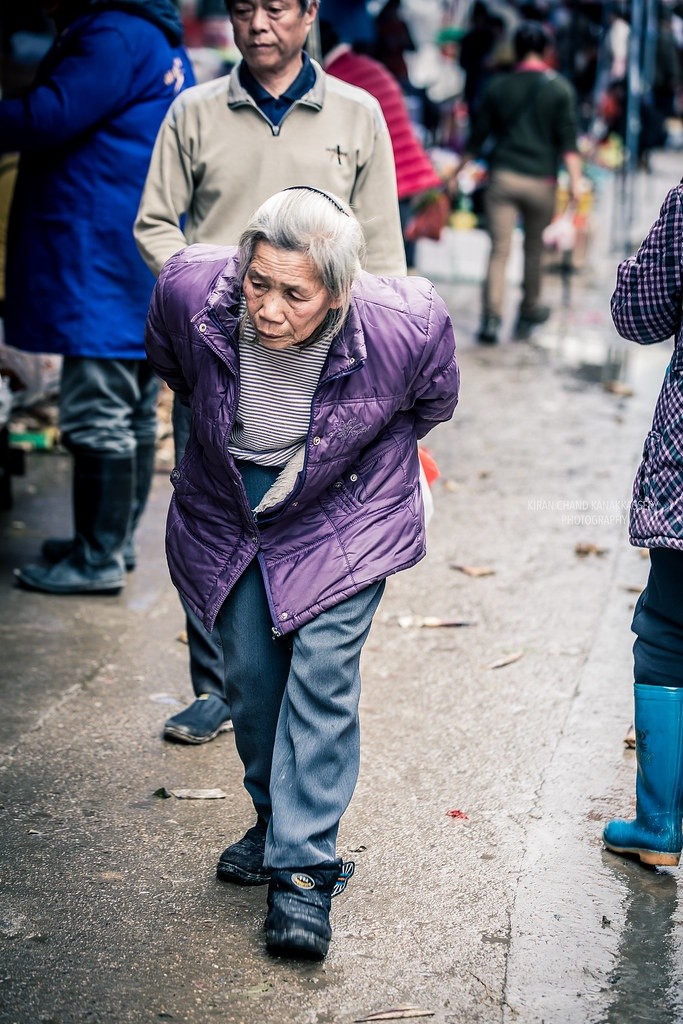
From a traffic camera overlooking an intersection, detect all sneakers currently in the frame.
[164,692,233,745]
[263,856,355,961]
[217,818,271,886]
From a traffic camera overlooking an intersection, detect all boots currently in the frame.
[601,683,683,866]
[12,446,137,595]
[42,443,156,572]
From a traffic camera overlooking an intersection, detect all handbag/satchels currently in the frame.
[471,184,489,213]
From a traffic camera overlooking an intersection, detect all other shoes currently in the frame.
[517,304,551,339]
[476,317,501,342]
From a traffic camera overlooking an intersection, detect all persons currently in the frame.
[131,0,409,746]
[463,20,576,348]
[313,8,441,269]
[374,0,680,157]
[603,163,683,870]
[138,183,461,952]
[2,0,199,598]
